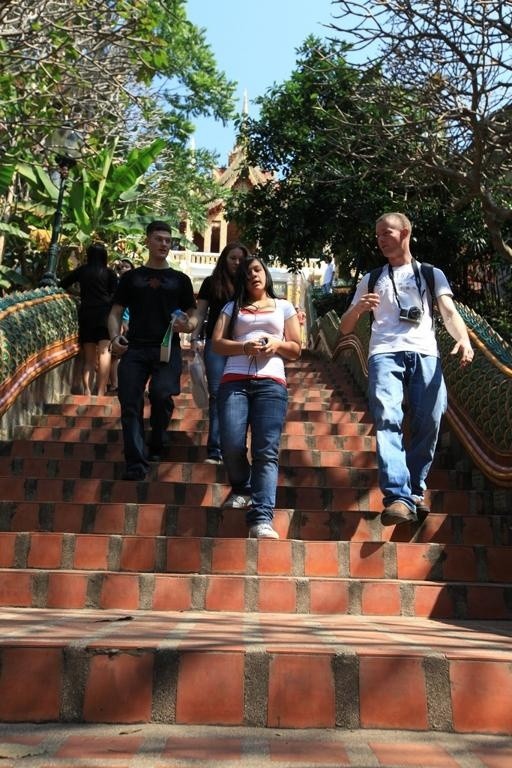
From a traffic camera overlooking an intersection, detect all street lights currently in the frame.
[39,116,84,290]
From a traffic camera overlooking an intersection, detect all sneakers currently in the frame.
[145,427,161,446]
[203,457,224,465]
[224,495,252,509]
[248,524,279,539]
[380,490,431,526]
[121,470,144,480]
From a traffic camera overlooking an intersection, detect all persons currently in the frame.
[320,255,335,294]
[108,222,197,480]
[294,305,306,342]
[62,243,118,396]
[190,243,248,464]
[109,259,135,391]
[340,212,474,525]
[212,256,300,538]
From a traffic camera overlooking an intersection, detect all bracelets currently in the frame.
[190,338,199,342]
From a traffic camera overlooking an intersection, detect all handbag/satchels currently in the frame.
[190,352,210,411]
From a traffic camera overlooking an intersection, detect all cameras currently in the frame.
[258,337,268,345]
[116,266,122,271]
[400,305,424,324]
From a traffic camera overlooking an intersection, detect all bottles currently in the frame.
[172,309,183,328]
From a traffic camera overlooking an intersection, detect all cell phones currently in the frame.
[119,338,128,346]
